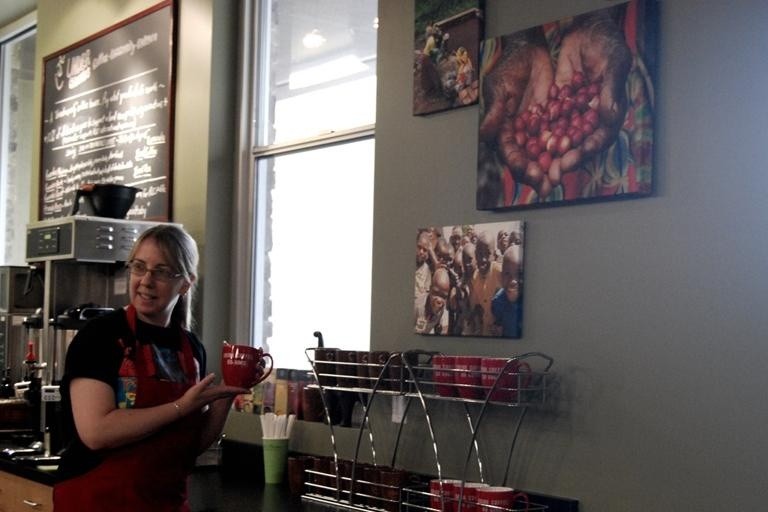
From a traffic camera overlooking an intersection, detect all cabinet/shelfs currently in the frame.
[299,347,580,512]
[0,469,53,512]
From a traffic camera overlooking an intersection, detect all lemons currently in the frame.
[71,184,143,219]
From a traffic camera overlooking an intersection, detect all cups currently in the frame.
[129,261,183,282]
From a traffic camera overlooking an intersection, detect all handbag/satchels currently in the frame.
[173,401,183,416]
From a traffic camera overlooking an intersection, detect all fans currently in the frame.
[39,1,173,223]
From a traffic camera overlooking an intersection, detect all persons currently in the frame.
[415,225,521,339]
[422,21,474,102]
[50,225,250,510]
[477,9,636,196]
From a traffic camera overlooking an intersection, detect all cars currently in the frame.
[220,344,274,388]
[262,438,288,484]
[433,354,532,404]
[301,387,325,421]
[288,455,421,512]
[314,347,418,392]
[429,478,530,512]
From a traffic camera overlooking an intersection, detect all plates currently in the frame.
[0,469,53,512]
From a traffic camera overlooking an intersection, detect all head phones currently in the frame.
[262,413,294,438]
